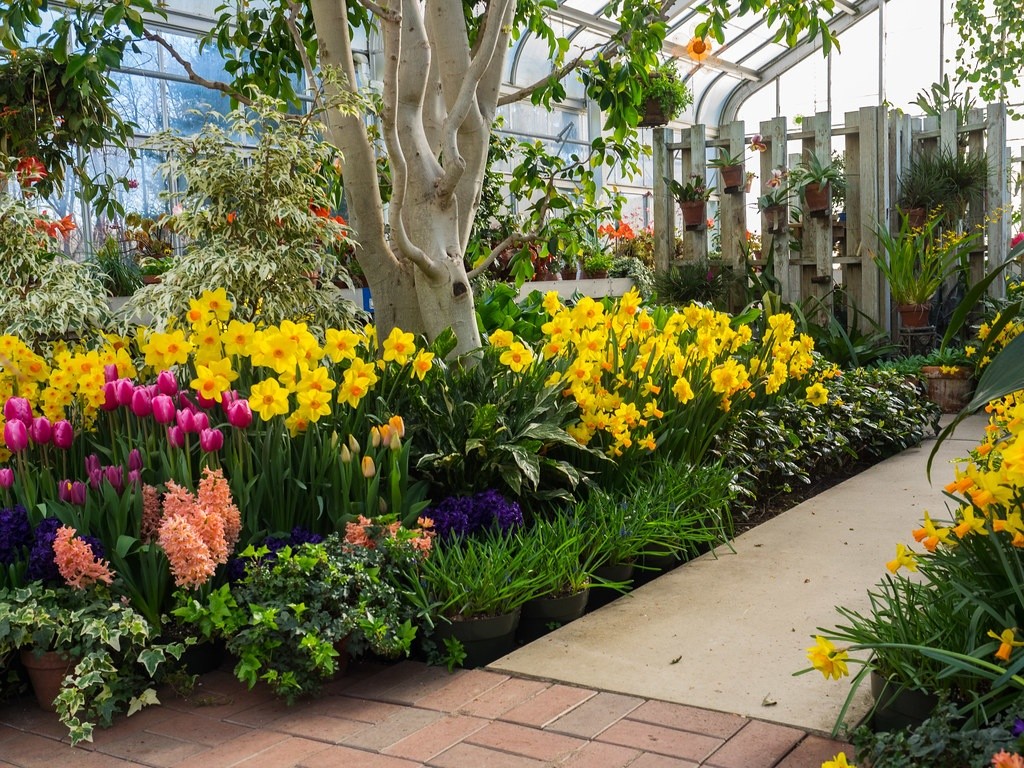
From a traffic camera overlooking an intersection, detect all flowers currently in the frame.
[740,169,806,217]
[704,133,768,169]
[0,202,1024,768]
[662,163,718,203]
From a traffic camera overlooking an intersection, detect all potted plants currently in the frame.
[629,63,693,128]
[745,171,759,194]
[786,544,984,739]
[509,500,634,645]
[629,450,737,573]
[895,138,1001,232]
[560,263,578,280]
[681,453,759,559]
[0,578,199,749]
[416,526,569,664]
[866,355,925,403]
[799,146,847,212]
[579,486,681,599]
[580,252,614,279]
[139,256,174,285]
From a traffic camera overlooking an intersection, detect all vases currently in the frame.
[680,201,705,226]
[753,250,763,272]
[763,206,786,228]
[920,365,974,412]
[892,303,932,327]
[132,633,225,675]
[720,165,744,188]
